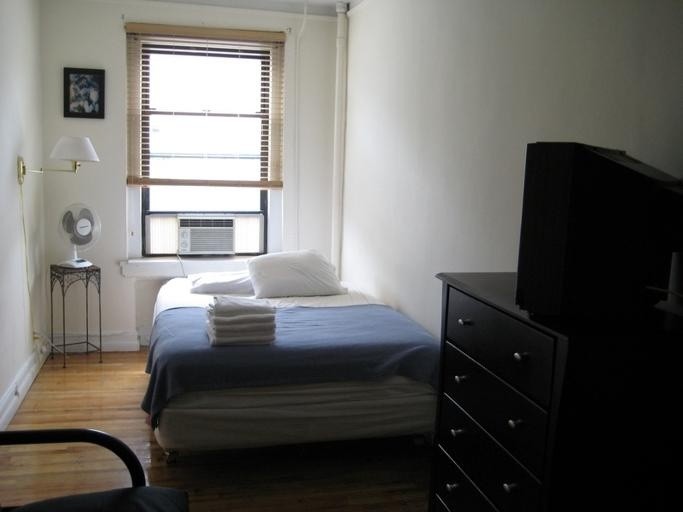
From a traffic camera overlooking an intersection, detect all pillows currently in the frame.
[187,270,253,294]
[244,246,348,298]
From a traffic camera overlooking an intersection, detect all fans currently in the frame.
[56,202,102,270]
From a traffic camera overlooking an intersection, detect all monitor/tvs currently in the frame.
[516,141,682,316]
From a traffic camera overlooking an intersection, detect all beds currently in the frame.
[151,278,442,469]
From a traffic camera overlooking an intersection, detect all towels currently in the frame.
[205,296,277,347]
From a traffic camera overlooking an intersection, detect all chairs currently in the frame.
[0,426,190,511]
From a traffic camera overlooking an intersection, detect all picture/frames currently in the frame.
[63,66,106,120]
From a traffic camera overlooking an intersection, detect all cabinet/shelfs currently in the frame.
[428,271,683,511]
[49,263,102,369]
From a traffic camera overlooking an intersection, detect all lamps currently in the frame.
[17,133,101,187]
